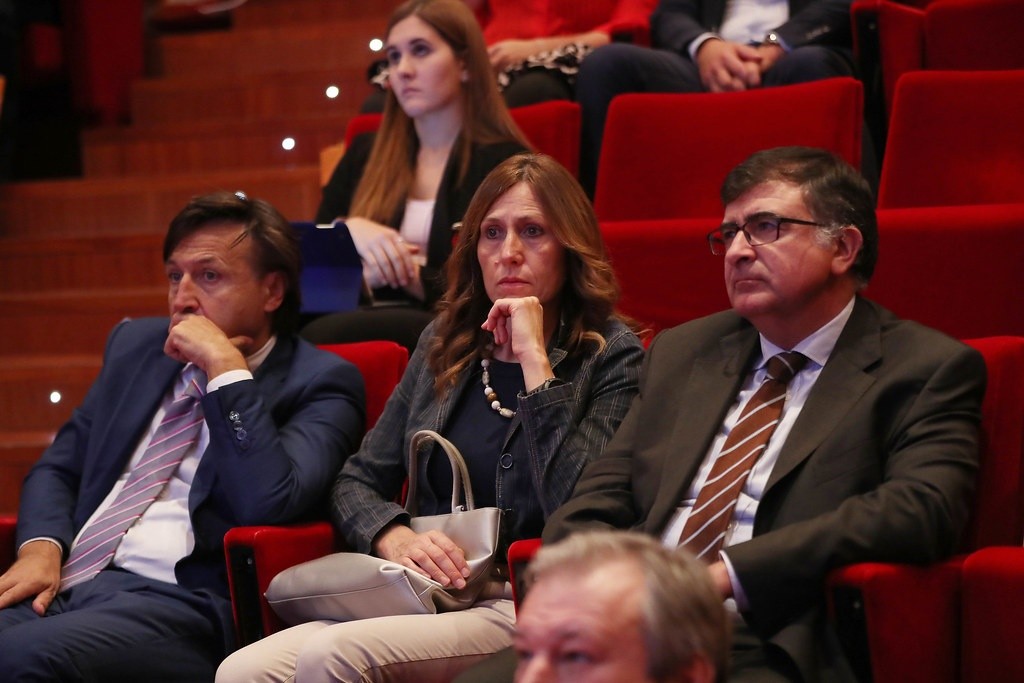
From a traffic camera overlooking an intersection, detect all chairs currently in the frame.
[0,0,1024,683]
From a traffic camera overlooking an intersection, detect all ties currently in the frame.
[678,351,808,566]
[58,367,208,591]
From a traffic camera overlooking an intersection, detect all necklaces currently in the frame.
[479,337,519,419]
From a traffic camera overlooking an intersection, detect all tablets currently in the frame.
[291,222,374,306]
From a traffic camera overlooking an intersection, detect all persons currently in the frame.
[286,0,530,345]
[360,0,657,115]
[0,144,987,683]
[570,0,851,204]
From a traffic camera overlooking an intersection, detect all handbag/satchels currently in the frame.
[264,431,508,614]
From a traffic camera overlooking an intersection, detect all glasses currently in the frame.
[707,212,838,256]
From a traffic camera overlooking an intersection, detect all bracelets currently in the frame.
[764,30,791,54]
